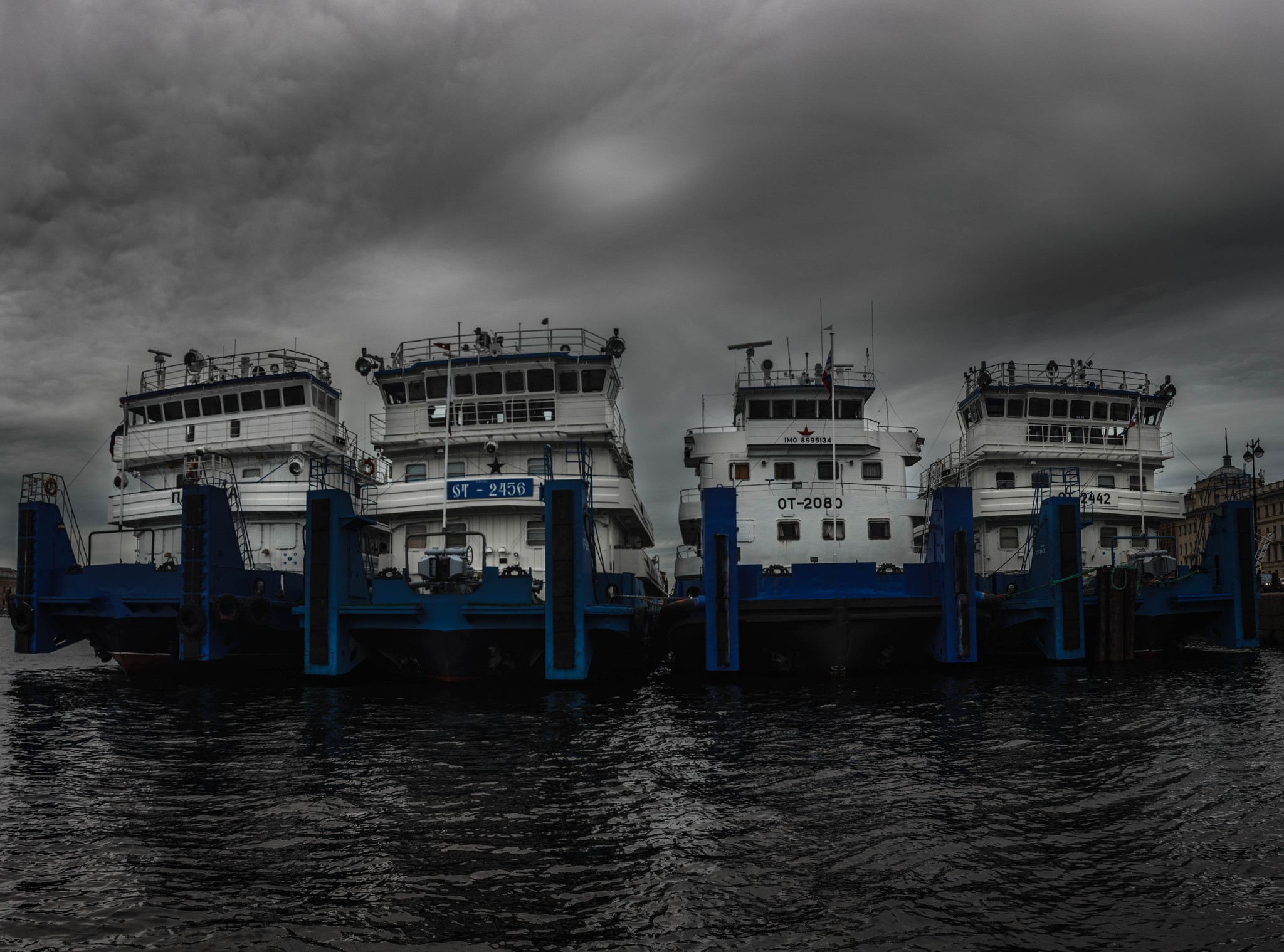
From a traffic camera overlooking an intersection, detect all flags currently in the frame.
[821,336,833,402]
[444,358,454,436]
[1116,400,1138,437]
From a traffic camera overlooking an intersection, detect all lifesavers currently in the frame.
[176,604,205,634]
[242,597,272,627]
[212,594,240,622]
[45,478,57,496]
[363,460,375,475]
[1047,362,1057,373]
[10,605,34,633]
[190,462,202,482]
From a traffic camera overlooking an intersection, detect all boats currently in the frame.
[4,293,1262,678]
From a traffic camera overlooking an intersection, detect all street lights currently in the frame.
[1243,437,1264,593]
